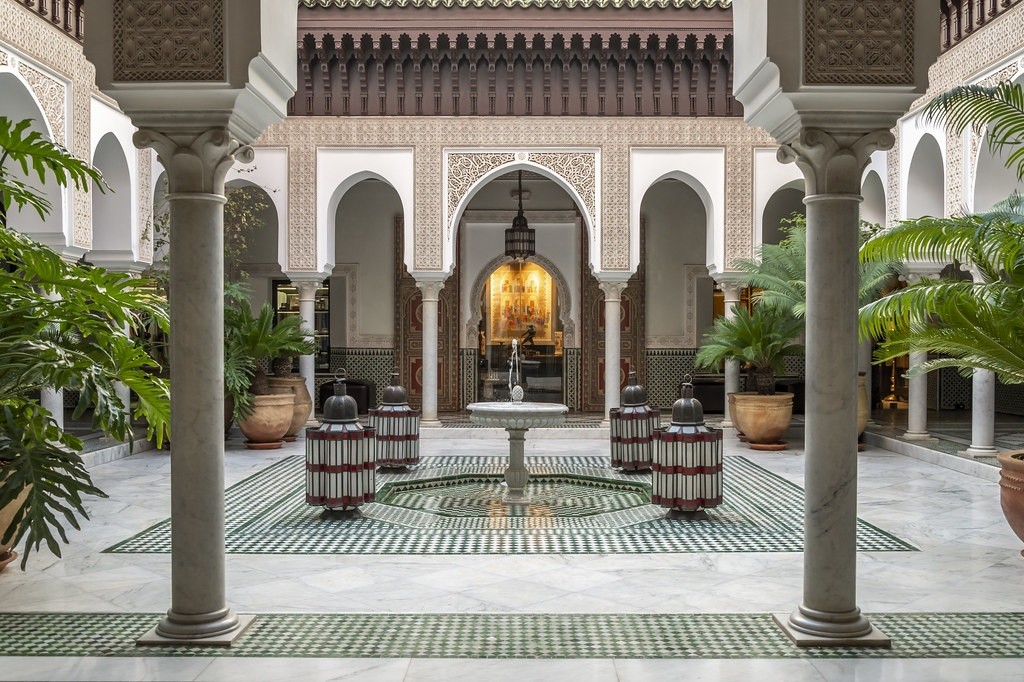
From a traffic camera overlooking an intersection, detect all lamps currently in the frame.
[505,170,535,262]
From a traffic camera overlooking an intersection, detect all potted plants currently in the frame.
[0,117,314,572]
[693,78,1024,558]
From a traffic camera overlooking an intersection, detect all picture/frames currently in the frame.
[486,262,556,346]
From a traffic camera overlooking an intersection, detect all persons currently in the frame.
[521,325,536,345]
